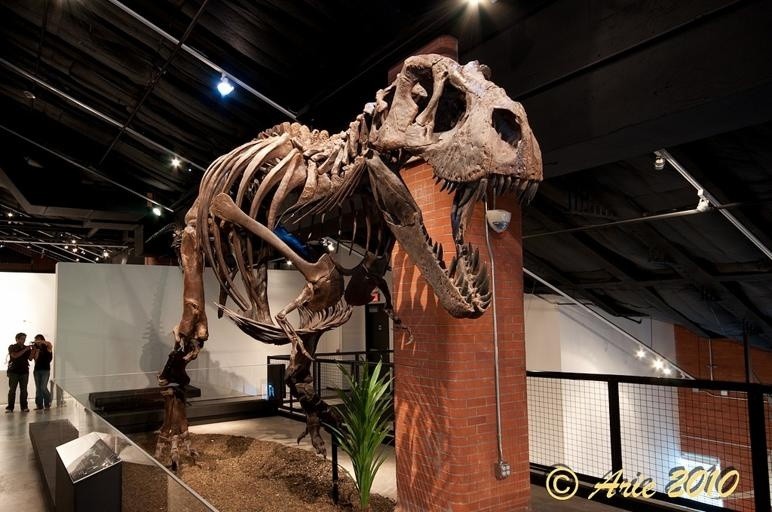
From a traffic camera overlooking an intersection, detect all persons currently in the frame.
[34,335,52,410]
[6,333,35,412]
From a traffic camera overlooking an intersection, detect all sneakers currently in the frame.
[34,406,42,410]
[44,407,50,411]
[5,409,13,412]
[21,407,28,411]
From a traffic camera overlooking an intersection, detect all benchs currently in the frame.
[30,418,79,507]
